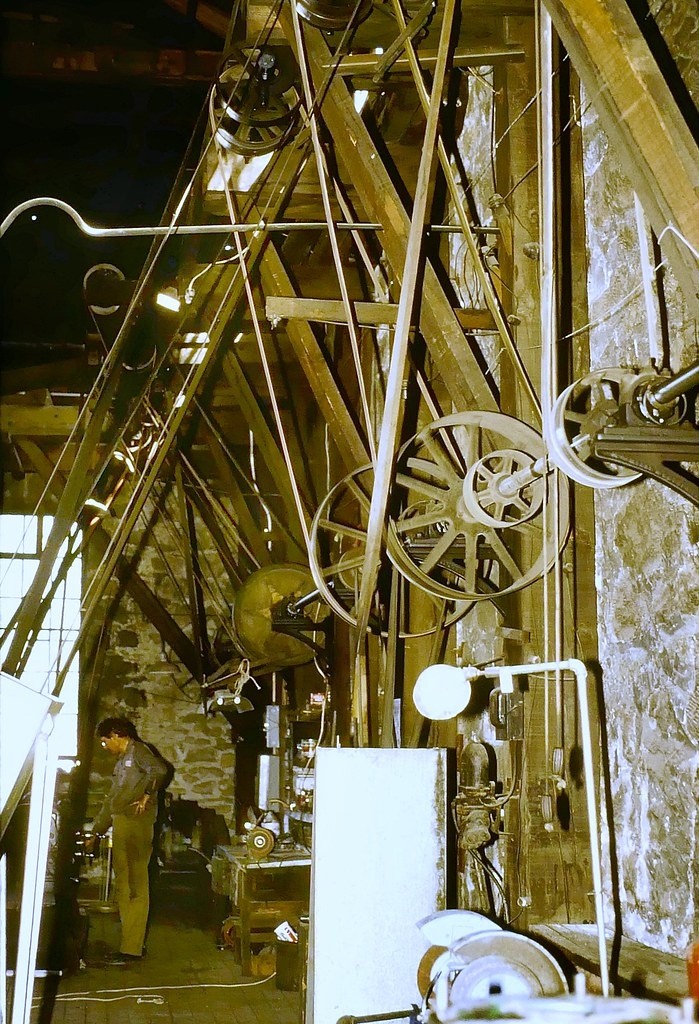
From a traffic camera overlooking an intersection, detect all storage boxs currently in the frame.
[211,857,287,926]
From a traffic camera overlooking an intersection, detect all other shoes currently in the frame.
[106,946,147,965]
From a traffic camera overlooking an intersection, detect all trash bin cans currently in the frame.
[275,939,300,992]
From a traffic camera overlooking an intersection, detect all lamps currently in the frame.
[155,285,180,313]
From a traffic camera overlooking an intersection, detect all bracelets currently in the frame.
[145,790,151,795]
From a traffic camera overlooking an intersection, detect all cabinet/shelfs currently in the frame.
[216,720,332,976]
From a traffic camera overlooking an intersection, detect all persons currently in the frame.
[84,718,167,964]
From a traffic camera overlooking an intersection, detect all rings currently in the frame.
[140,805,142,808]
